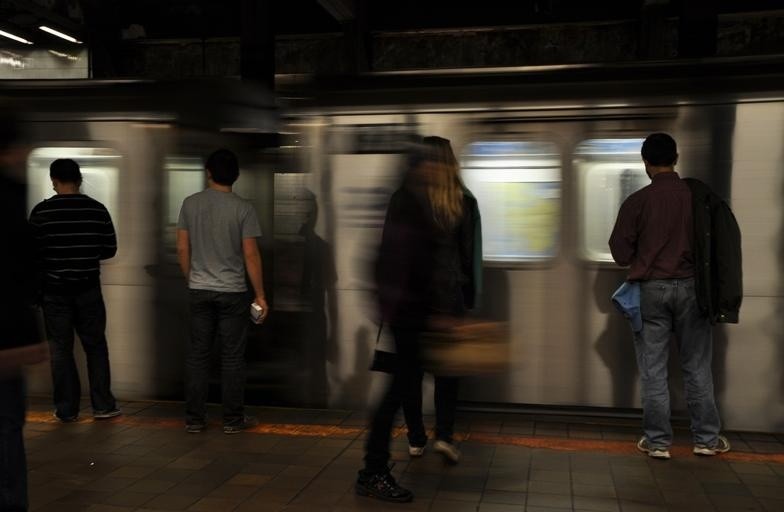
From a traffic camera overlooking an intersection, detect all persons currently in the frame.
[609,133,730,459]
[352,132,507,504]
[176,150,269,434]
[401,136,482,461]
[26,159,122,423]
[0,119,50,512]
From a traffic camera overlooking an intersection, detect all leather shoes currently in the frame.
[355,467,414,502]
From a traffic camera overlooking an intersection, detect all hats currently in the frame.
[612,281,643,331]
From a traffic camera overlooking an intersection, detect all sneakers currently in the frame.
[185,420,208,433]
[432,440,460,463]
[408,444,424,456]
[693,436,730,455]
[93,409,121,418]
[637,436,670,459]
[53,409,79,423]
[223,416,257,434]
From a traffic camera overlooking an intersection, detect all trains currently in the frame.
[12,58,784,430]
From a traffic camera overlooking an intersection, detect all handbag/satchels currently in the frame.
[370,349,400,374]
